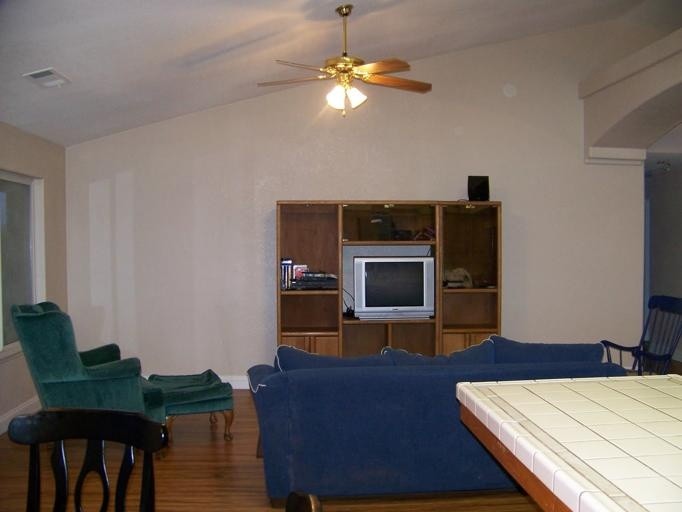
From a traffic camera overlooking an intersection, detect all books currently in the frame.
[281,265,292,288]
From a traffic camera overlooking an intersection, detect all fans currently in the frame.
[256,5,432,119]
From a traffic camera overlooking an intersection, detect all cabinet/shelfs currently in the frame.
[276,200,502,357]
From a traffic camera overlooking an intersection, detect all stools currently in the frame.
[148,369,233,447]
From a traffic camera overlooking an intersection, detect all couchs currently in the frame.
[248,362,628,512]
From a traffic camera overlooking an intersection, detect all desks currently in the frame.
[454,373,682,511]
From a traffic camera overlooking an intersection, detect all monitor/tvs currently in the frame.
[353,256,435,321]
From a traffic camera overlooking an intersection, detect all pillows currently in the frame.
[380,340,496,364]
[490,334,604,361]
[274,344,393,372]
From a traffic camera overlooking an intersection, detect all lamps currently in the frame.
[326,7,367,112]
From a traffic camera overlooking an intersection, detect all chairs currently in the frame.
[10,301,166,462]
[600,294,682,375]
[9,406,169,511]
